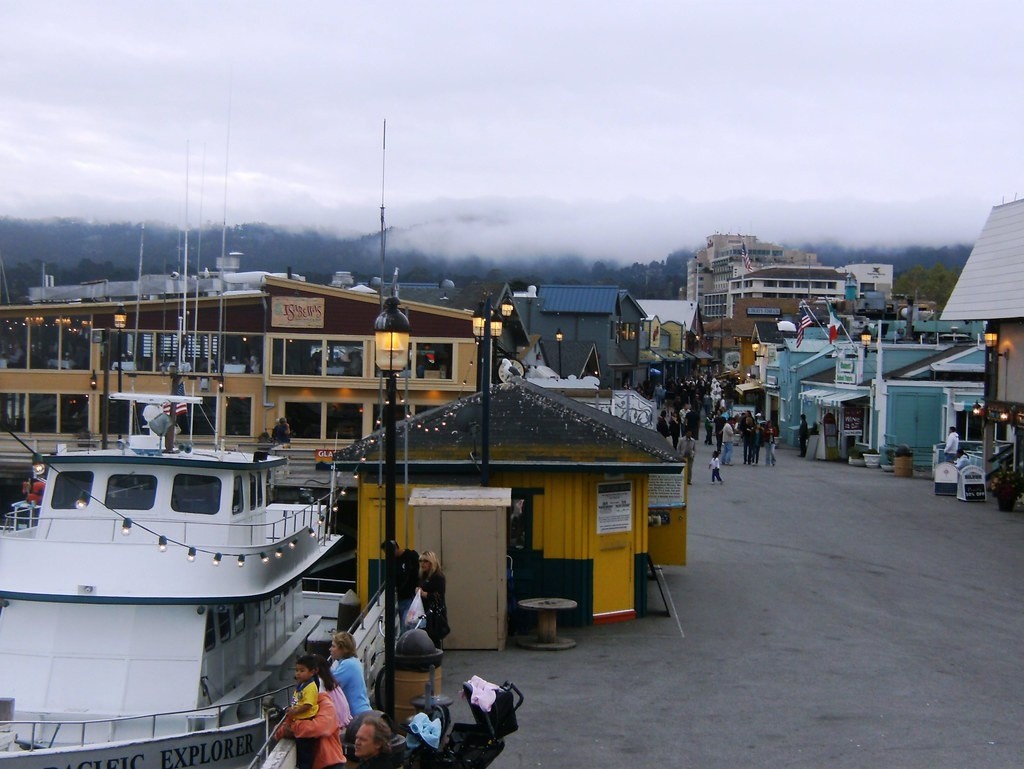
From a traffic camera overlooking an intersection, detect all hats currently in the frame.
[755,413,761,416]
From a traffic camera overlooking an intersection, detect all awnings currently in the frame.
[736,383,764,395]
[798,386,869,407]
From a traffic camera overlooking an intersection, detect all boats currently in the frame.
[0,84,356,769]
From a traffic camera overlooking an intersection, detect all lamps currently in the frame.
[89,369,100,392]
[972,400,1010,423]
[983,327,1009,364]
[752,338,764,360]
[860,326,877,359]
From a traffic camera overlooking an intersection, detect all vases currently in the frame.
[996,484,1019,511]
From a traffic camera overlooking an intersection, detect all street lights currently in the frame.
[471,296,503,488]
[113,302,127,440]
[372,294,410,726]
[555,328,563,379]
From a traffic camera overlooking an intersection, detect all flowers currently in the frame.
[990,460,1024,498]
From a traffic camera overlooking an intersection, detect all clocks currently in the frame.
[498,359,525,383]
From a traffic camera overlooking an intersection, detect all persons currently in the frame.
[416,354,434,378]
[210,354,262,374]
[6,344,88,368]
[306,351,321,375]
[346,351,362,376]
[709,451,724,484]
[944,427,969,470]
[620,370,777,484]
[272,417,291,444]
[274,632,371,769]
[355,717,403,769]
[799,414,809,457]
[382,540,450,648]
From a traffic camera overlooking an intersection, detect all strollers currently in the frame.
[398,674,524,769]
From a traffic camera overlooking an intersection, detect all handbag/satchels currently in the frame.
[405,588,426,629]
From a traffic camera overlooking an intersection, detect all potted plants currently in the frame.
[848,444,914,476]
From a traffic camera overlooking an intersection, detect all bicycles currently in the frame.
[373,612,427,713]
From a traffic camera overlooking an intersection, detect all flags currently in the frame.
[742,243,752,272]
[796,307,812,349]
[828,305,841,344]
[163,382,187,416]
